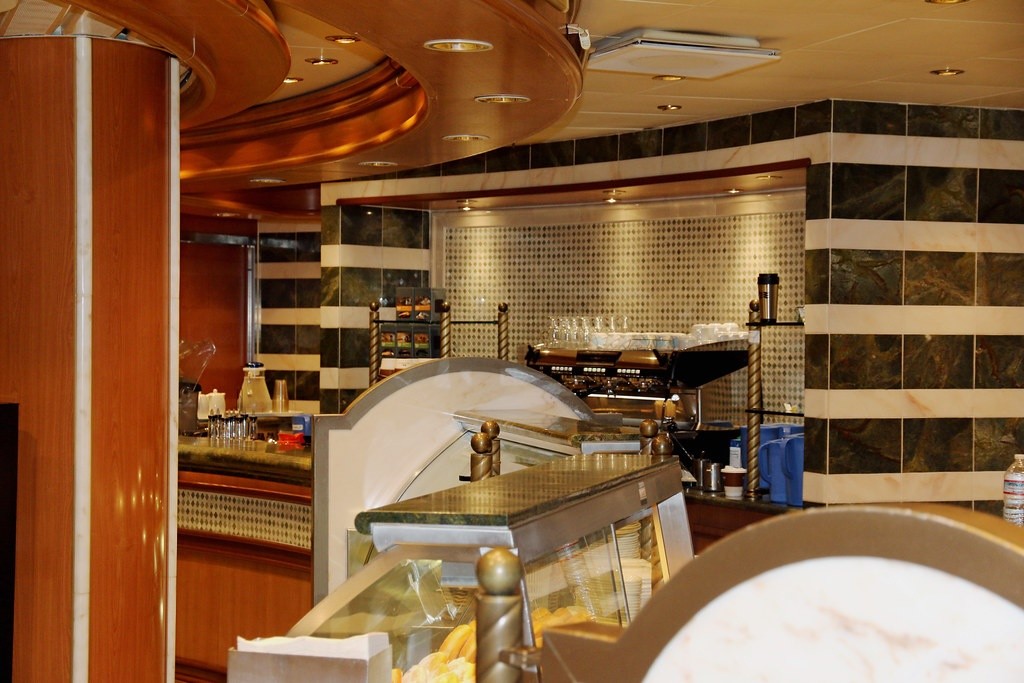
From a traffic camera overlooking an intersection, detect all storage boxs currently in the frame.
[377,287,443,358]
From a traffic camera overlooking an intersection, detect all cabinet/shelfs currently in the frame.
[348,409,697,683]
[744,299,804,500]
[529,348,702,434]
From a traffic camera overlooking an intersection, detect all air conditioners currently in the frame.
[585,29,782,80]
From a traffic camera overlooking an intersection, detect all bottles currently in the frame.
[547,316,631,350]
[1003,454,1024,528]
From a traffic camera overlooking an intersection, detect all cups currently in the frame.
[757,273,779,322]
[593,322,749,350]
[207,414,258,442]
[721,469,747,497]
[272,379,289,412]
